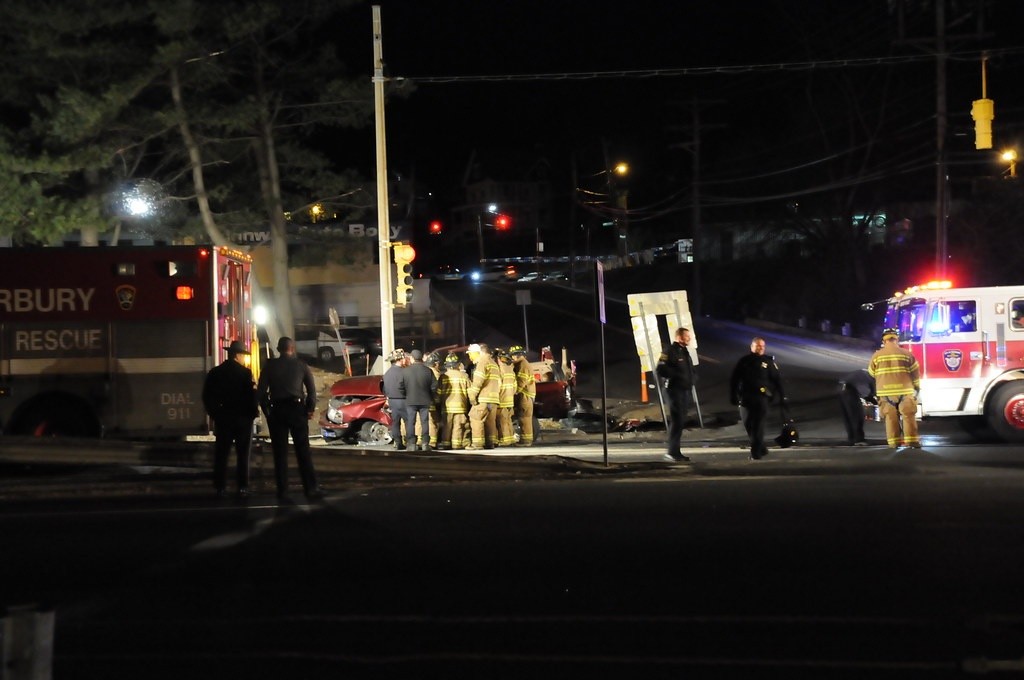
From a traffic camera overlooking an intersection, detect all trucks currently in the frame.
[0,237,261,442]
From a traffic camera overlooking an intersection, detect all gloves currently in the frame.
[468,392,478,406]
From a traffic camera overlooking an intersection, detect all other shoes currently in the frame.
[664,452,691,462]
[891,445,897,448]
[238,489,248,496]
[306,490,329,498]
[216,489,224,496]
[907,445,921,449]
[394,438,535,453]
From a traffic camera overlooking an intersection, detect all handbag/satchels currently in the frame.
[774,423,799,448]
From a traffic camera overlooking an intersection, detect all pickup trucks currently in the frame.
[317,342,578,447]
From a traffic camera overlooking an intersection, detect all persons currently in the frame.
[256,336,329,500]
[203,341,259,496]
[383,349,442,451]
[731,338,786,461]
[868,330,922,448]
[656,328,694,462]
[437,342,536,451]
[839,369,876,445]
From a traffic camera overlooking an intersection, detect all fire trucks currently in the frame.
[859,280,1024,445]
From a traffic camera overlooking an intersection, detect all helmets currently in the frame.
[491,347,512,360]
[466,343,480,353]
[425,352,443,363]
[478,343,489,353]
[445,353,460,366]
[882,328,899,340]
[384,349,407,361]
[509,345,525,356]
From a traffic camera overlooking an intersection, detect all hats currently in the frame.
[408,350,422,359]
[222,341,251,355]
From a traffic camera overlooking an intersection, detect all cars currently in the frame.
[470,264,568,285]
[293,322,559,382]
[430,264,466,283]
